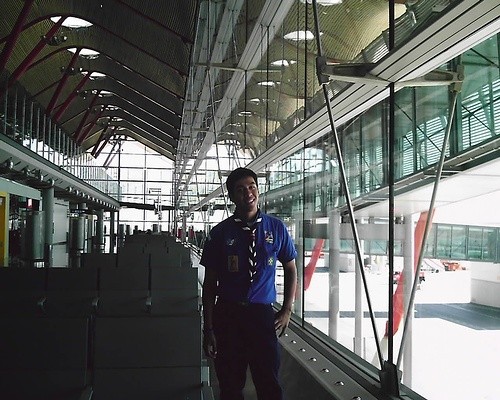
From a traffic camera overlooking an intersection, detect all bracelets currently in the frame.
[202,327,213,333]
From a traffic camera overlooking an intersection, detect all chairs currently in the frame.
[1,230,209,399]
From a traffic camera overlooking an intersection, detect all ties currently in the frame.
[245,227,257,284]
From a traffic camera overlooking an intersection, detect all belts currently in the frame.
[219,295,275,309]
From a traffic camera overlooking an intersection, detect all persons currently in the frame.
[198,167,299,400]
